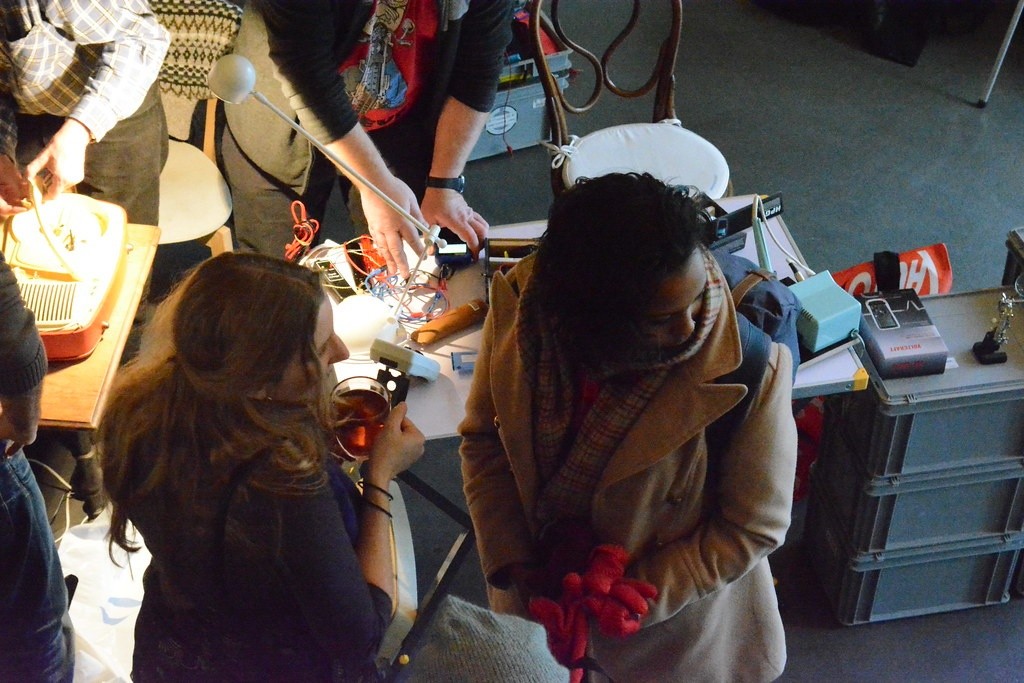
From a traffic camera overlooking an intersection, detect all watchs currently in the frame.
[424,175,465,193]
[88,129,96,143]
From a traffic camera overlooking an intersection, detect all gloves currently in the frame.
[531,543,659,683]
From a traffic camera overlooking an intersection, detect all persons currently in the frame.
[459,172,796,683]
[0,0,518,683]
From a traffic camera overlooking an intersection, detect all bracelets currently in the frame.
[361,499,393,518]
[358,480,393,503]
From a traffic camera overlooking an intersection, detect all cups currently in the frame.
[331,375,391,461]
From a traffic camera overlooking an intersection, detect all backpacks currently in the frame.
[710,248,803,455]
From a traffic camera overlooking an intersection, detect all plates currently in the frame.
[11,193,112,247]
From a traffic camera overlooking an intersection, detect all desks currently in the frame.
[328,194,868,683]
[38,224,161,523]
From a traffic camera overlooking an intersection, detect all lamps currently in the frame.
[206,55,448,351]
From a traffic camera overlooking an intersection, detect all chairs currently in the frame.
[150,0,243,255]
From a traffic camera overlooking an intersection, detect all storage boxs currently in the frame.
[464,9,573,161]
[853,288,948,380]
[802,287,1024,625]
